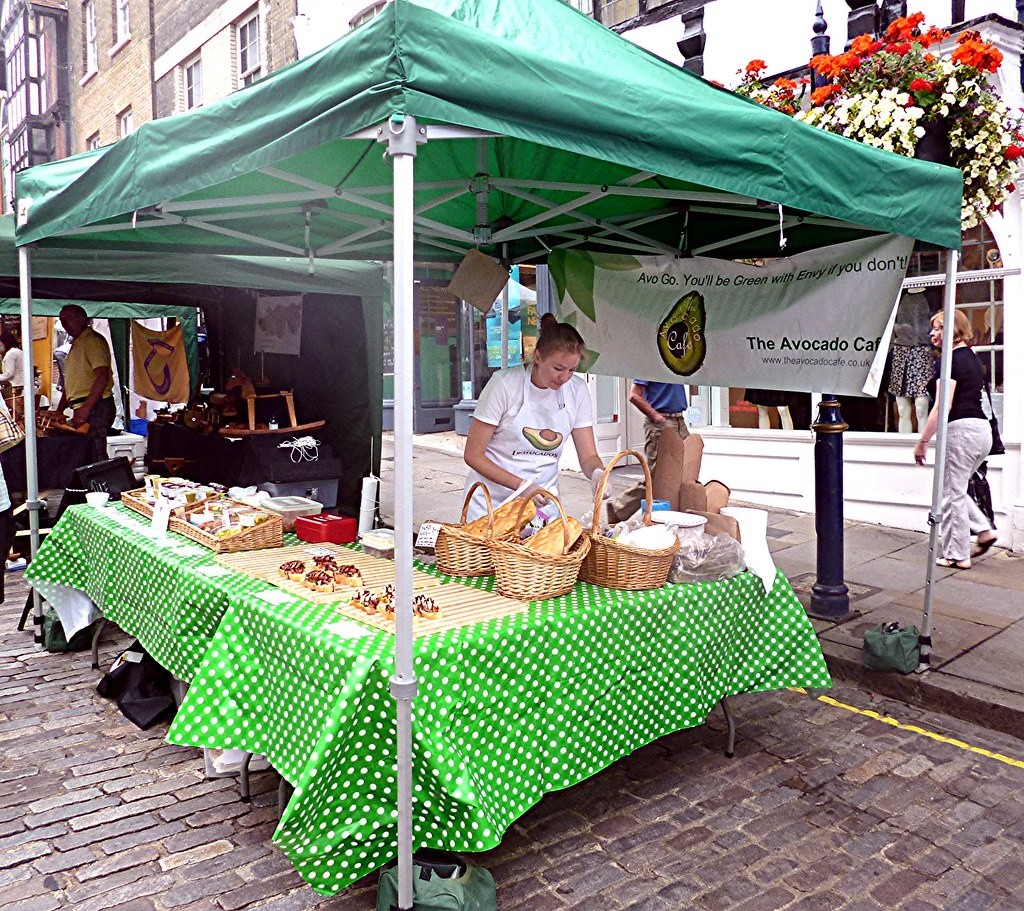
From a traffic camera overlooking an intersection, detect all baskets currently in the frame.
[122,486,221,524]
[169,497,283,554]
[575,450,680,590]
[423,483,514,578]
[486,491,591,602]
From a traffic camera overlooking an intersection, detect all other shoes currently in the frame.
[607,500,620,524]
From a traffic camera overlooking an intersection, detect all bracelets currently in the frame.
[920,439,928,443]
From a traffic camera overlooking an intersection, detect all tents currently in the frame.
[12,0,960,911]
[0,213,385,513]
[0,298,199,404]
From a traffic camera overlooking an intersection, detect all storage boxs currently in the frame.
[259,496,324,533]
[296,512,356,545]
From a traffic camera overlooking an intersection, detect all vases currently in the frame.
[911,121,951,165]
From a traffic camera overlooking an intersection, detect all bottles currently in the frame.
[269,418,278,430]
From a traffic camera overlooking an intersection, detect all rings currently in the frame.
[81,420,83,422]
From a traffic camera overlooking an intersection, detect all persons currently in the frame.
[54,344,73,391]
[887,286,942,433]
[629,379,690,471]
[742,389,794,429]
[56,305,117,488]
[914,309,999,568]
[459,313,611,521]
[0,331,24,396]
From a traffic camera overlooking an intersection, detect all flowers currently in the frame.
[787,12,1024,230]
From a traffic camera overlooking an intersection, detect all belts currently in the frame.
[658,413,683,417]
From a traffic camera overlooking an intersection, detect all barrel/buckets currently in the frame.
[123,419,148,437]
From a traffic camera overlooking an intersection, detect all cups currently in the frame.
[131,475,214,509]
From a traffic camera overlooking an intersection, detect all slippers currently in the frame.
[936,556,971,569]
[971,537,997,558]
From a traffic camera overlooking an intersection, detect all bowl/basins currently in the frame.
[652,511,707,532]
[261,496,323,532]
[204,499,269,538]
[359,529,395,558]
[5,394,41,413]
[86,492,109,507]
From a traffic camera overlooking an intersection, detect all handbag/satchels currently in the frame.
[0,407,25,453]
[862,622,919,674]
[968,461,998,535]
[44,607,92,652]
[988,418,1005,455]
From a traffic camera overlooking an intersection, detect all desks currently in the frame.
[21,501,833,895]
